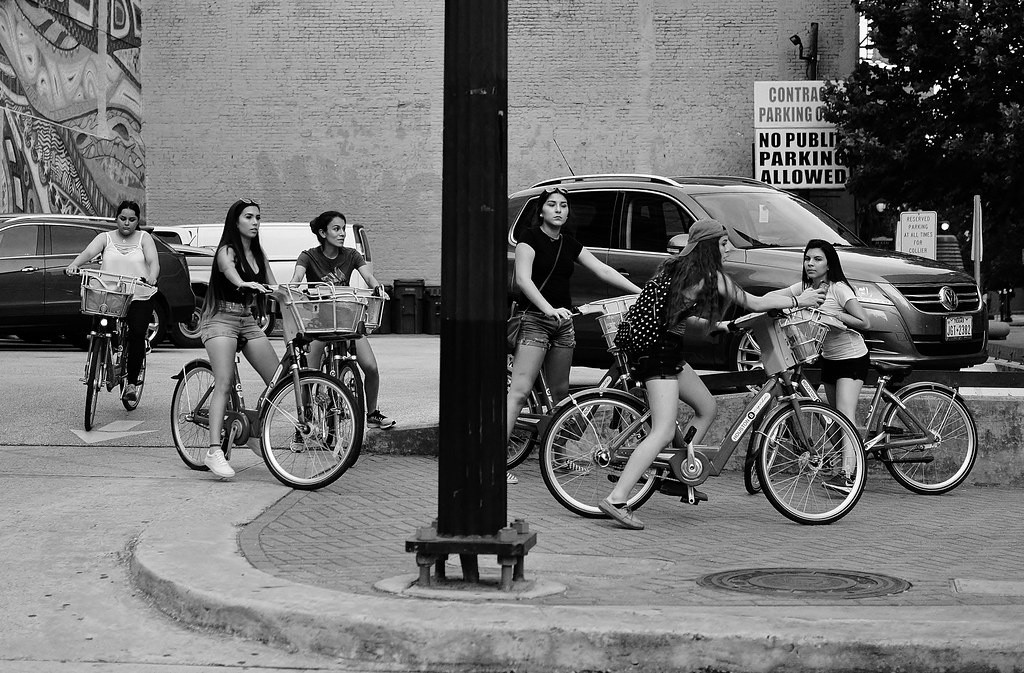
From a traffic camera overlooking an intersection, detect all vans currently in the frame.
[166,221,373,338]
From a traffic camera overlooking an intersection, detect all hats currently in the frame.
[678,220,729,256]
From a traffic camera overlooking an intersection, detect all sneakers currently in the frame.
[367,406,397,430]
[245,436,263,457]
[821,467,856,494]
[204,449,235,478]
[302,405,319,427]
[800,456,834,477]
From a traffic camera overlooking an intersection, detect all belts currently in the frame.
[219,301,253,315]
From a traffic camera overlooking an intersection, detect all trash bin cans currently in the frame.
[424,286,442,335]
[393,278,425,334]
[369,285,393,334]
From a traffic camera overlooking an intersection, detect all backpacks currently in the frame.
[612,266,696,358]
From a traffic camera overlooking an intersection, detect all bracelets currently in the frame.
[793,296,799,309]
[790,296,794,309]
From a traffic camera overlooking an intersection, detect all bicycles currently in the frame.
[171,280,369,490]
[304,282,390,465]
[63,267,148,431]
[505,293,980,527]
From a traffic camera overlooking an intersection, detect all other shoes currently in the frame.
[554,460,590,476]
[660,472,708,501]
[598,498,644,529]
[506,468,518,483]
[126,385,137,402]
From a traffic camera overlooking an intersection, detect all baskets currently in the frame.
[81,269,139,318]
[779,304,832,366]
[278,281,365,342]
[319,281,385,335]
[595,310,627,353]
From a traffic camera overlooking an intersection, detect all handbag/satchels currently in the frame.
[88,272,127,314]
[507,316,522,353]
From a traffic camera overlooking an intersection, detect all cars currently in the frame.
[164,240,219,349]
[142,224,194,247]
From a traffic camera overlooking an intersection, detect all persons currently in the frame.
[507,184,641,483]
[288,211,396,453]
[66,199,160,400]
[203,197,280,477]
[599,218,826,530]
[762,239,871,493]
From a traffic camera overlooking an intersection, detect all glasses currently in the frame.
[544,185,569,195]
[238,196,263,206]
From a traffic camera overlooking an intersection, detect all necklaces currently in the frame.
[115,229,129,244]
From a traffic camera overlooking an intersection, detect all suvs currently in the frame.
[509,172,987,386]
[0,213,196,350]
